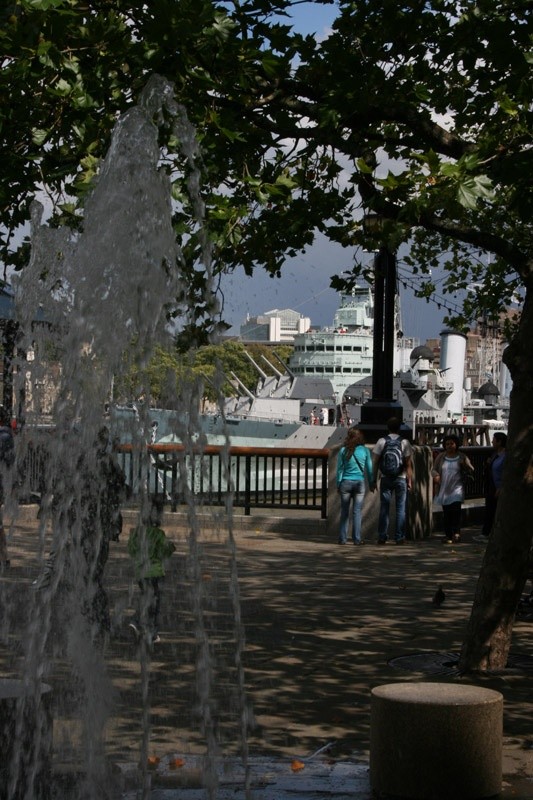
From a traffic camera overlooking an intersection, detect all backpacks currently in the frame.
[379,436,407,477]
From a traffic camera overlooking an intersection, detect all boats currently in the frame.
[104,281,509,500]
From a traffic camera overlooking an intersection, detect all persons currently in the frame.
[335,426,376,545]
[372,417,413,545]
[125,503,177,645]
[472,433,507,543]
[0,404,15,574]
[66,427,131,632]
[430,434,475,544]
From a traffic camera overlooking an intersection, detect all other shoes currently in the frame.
[395,541,403,544]
[129,623,140,641]
[339,541,345,544]
[472,535,489,544]
[454,534,460,540]
[446,540,455,544]
[354,542,360,544]
[148,635,160,644]
[378,538,385,545]
